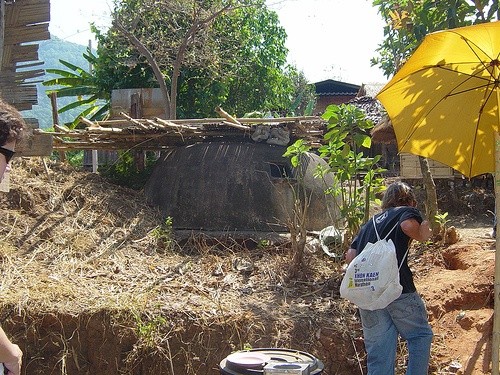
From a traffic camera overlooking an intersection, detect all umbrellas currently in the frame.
[371,19,500,181]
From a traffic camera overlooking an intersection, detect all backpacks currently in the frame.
[338,214,413,311]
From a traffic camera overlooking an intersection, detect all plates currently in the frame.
[226,352,271,368]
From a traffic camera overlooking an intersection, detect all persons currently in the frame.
[0,98,22,375]
[345,182,434,375]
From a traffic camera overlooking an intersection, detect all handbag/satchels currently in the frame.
[319,225,344,257]
